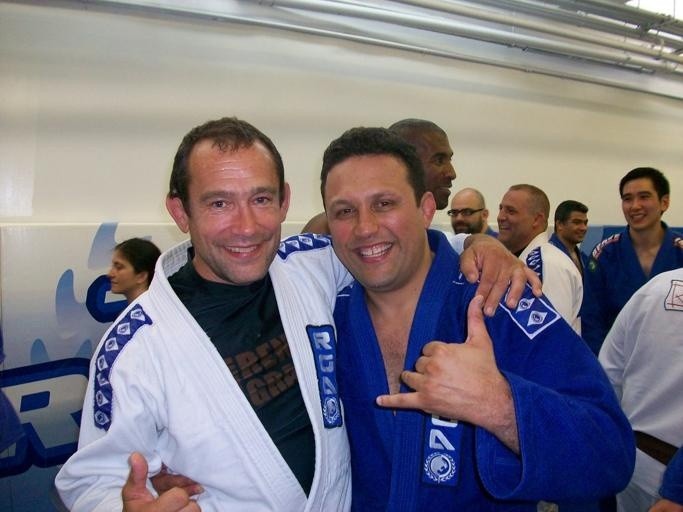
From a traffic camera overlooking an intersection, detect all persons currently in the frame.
[547,201,589,278]
[55,116,543,512]
[648,447,683,512]
[150,127,635,512]
[581,168,683,357]
[596,267,683,512]
[108,238,162,305]
[300,118,456,233]
[496,184,584,337]
[447,188,499,239]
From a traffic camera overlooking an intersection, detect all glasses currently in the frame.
[448,208,484,217]
